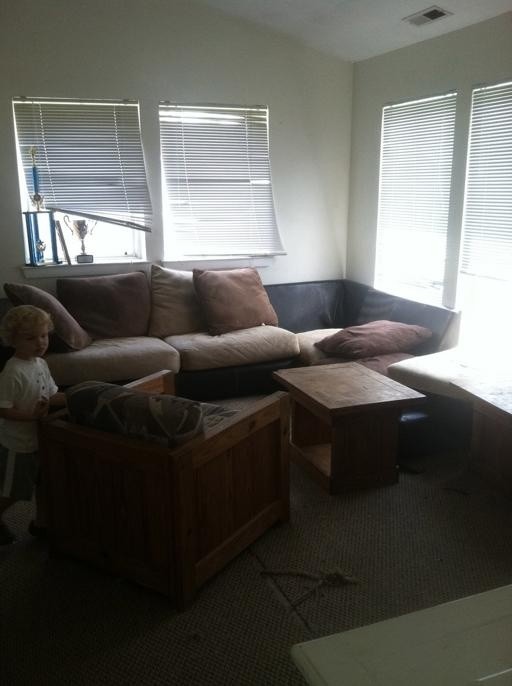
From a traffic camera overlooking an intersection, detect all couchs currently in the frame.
[0,279,460,404]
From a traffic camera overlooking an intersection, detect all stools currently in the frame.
[0,417,38,499]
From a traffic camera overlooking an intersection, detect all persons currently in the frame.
[0,305,68,551]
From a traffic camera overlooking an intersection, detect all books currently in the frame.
[54,219,72,266]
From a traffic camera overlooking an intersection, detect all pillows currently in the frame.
[314,319,432,359]
[4,264,279,352]
[69,380,241,448]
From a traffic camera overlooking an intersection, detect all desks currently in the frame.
[270,361,427,495]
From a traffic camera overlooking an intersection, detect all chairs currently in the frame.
[34,369,291,615]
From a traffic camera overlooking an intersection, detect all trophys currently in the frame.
[61,213,101,265]
